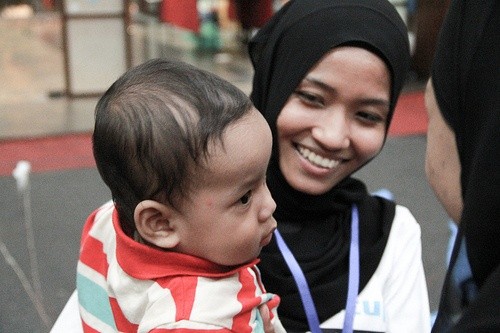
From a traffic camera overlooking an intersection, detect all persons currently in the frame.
[50,0,430,333]
[76,58,280,333]
[424,0,500,333]
